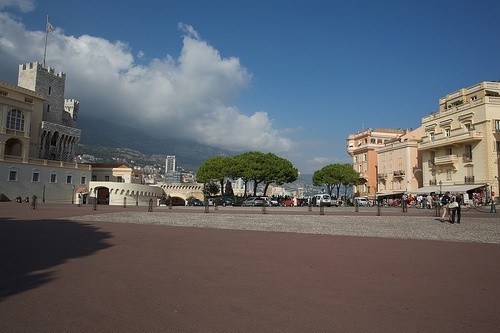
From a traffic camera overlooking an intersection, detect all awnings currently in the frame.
[376,189,407,207]
[417,185,489,207]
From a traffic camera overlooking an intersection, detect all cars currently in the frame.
[186,199,204,206]
[202,194,372,208]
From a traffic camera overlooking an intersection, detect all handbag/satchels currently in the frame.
[449,201,459,208]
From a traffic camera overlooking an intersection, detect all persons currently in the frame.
[271,194,350,207]
[31,194,37,211]
[402,194,408,212]
[441,191,452,223]
[471,189,495,208]
[376,193,439,210]
[450,192,462,224]
